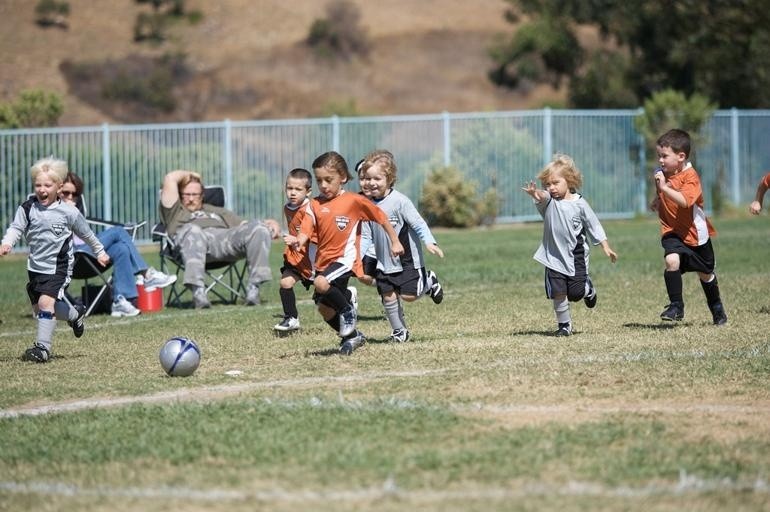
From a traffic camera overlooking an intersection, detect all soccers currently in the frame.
[159,336,200,377]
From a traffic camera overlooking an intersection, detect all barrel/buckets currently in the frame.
[135,283,163,312]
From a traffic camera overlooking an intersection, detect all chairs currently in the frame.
[148,184,264,308]
[23,188,145,321]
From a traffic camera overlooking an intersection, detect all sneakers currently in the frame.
[711,302,726,325]
[660,303,684,323]
[583,277,597,308]
[557,322,573,338]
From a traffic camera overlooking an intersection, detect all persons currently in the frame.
[283,149,405,355]
[156,171,281,312]
[271,167,359,334]
[522,154,618,337]
[355,159,378,288]
[749,172,769,214]
[0,155,110,365]
[56,172,178,318]
[360,149,447,348]
[650,128,729,327]
